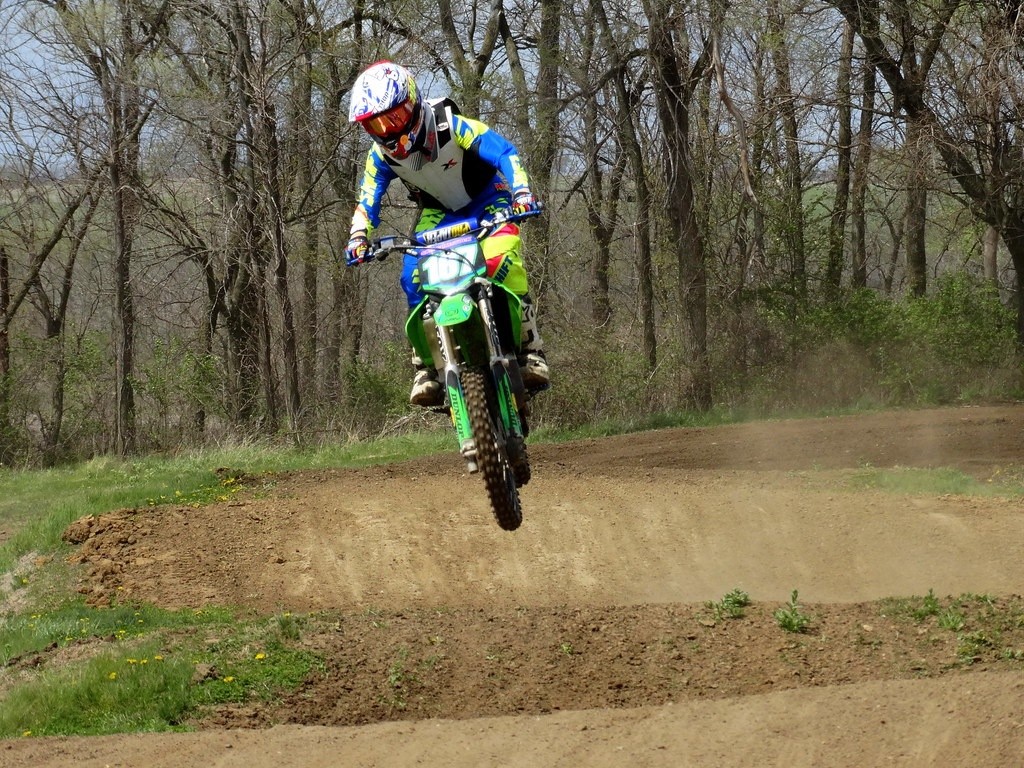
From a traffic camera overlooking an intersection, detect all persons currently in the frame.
[342,62,551,406]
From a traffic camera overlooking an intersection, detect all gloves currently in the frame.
[513,192,539,219]
[344,229,370,267]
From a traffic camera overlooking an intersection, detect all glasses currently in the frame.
[362,74,415,138]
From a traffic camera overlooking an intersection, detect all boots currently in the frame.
[410,347,445,407]
[516,292,549,390]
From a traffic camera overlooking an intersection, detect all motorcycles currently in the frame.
[345,200,550,532]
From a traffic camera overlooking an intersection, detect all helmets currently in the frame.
[349,65,423,160]
